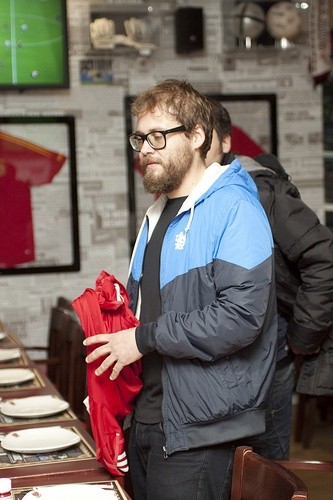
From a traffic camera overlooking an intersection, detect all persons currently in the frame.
[83,78,277,500]
[203,96,333,461]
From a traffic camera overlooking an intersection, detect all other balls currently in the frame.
[265,1,303,40]
[228,0,265,41]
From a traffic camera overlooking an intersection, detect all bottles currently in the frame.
[0,478,13,500]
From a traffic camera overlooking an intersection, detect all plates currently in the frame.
[0,397,70,418]
[0,350,21,361]
[22,483,119,500]
[0,428,81,453]
[0,332,6,340]
[0,370,35,384]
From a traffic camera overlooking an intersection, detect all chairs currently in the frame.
[231,446,308,500]
[45,297,87,418]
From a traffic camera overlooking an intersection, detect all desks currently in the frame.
[0,330,131,500]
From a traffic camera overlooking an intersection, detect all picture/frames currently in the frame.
[125,92,278,256]
[0,116,81,276]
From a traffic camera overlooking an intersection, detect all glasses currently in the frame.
[127,124,186,150]
